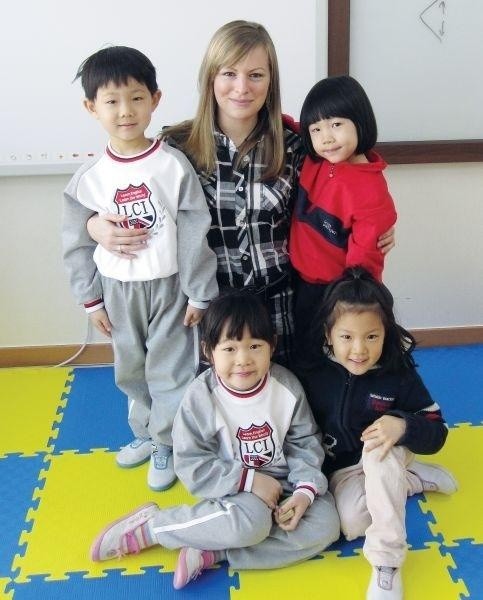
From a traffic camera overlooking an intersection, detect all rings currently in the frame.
[116,244,123,255]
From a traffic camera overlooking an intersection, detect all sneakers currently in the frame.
[89,500,159,563]
[405,460,458,496]
[171,545,207,590]
[146,442,179,491]
[114,438,151,469]
[364,564,402,600]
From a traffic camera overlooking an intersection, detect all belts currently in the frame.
[217,273,288,302]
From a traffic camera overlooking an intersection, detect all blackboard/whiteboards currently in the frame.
[328,0,483,165]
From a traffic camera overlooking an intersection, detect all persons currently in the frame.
[83,16,399,378]
[89,288,327,591]
[279,71,400,342]
[57,44,222,494]
[298,265,461,600]
